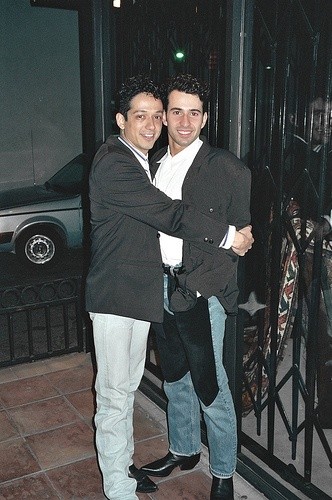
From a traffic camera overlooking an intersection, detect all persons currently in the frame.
[140,74,253,499]
[85,74,255,500]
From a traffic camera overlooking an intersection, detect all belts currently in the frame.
[163,264,180,275]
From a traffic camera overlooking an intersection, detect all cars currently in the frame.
[0,154,84,271]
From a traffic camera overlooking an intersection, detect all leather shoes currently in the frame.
[126,465,158,493]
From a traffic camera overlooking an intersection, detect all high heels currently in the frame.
[208,477,234,500]
[141,451,201,478]
